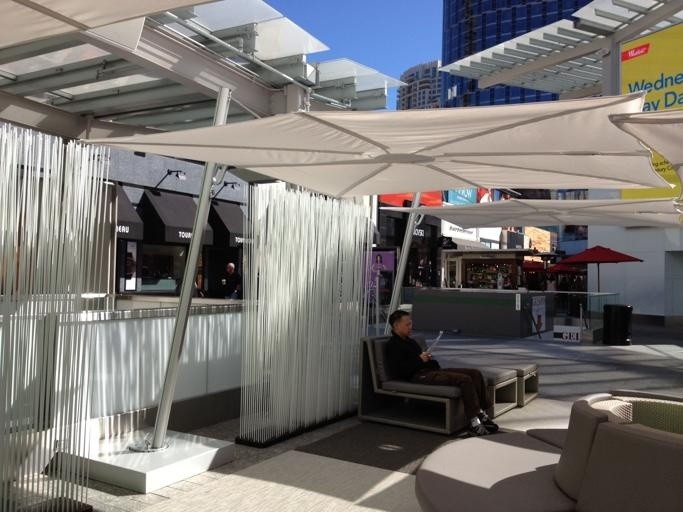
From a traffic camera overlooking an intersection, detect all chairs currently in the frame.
[357,333,539,436]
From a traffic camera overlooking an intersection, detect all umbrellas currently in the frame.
[522,244,643,292]
[605,107,683,191]
[73,86,677,449]
[377,190,683,335]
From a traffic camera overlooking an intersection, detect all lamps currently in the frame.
[151,169,241,205]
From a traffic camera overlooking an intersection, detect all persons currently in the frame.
[381,310,499,437]
[370,254,387,273]
[218,261,241,300]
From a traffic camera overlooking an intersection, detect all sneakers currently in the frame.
[468,417,490,436]
[480,414,498,433]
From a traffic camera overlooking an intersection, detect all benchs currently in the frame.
[415,389,682,510]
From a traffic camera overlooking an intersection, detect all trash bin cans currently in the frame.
[603,305,631,345]
